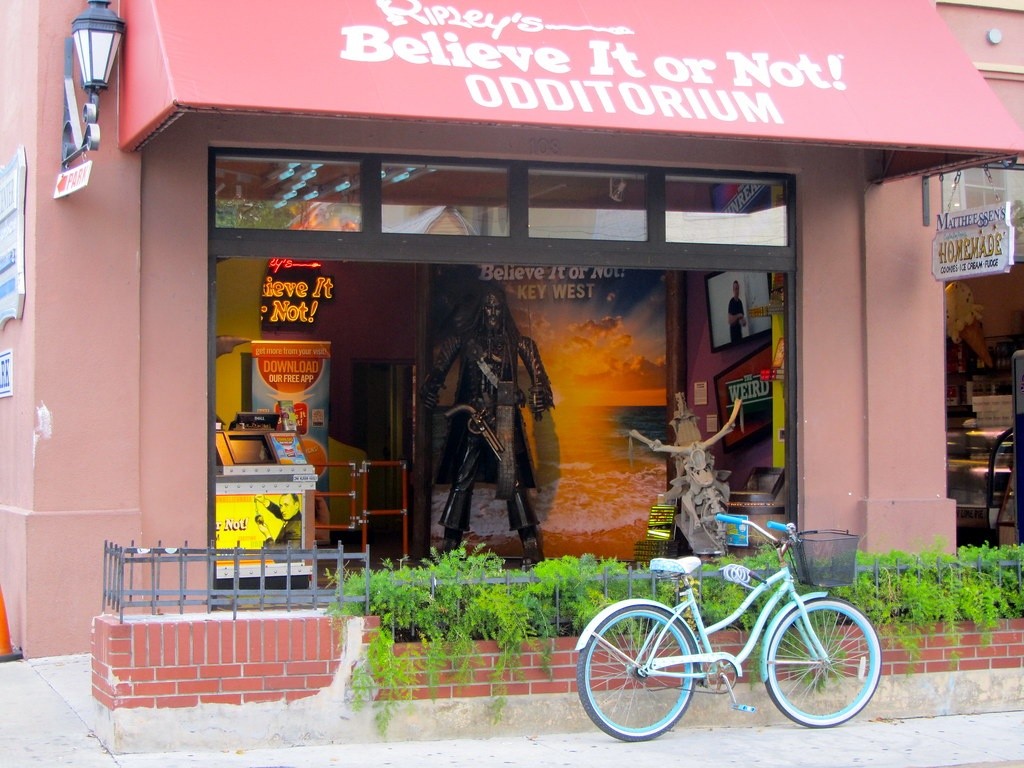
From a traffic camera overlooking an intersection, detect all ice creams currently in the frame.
[945,281,993,368]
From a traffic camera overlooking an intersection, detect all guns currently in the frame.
[442,404,505,463]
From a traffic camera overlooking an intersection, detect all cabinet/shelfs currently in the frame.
[946,404,1018,546]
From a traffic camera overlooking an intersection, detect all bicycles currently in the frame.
[570,512,882,743]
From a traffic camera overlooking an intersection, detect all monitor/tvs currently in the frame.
[224,430,277,463]
[228,412,280,431]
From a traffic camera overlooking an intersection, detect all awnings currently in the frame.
[118,0,1023,186]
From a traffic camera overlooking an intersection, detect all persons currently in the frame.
[727,280,745,342]
[421,278,556,573]
